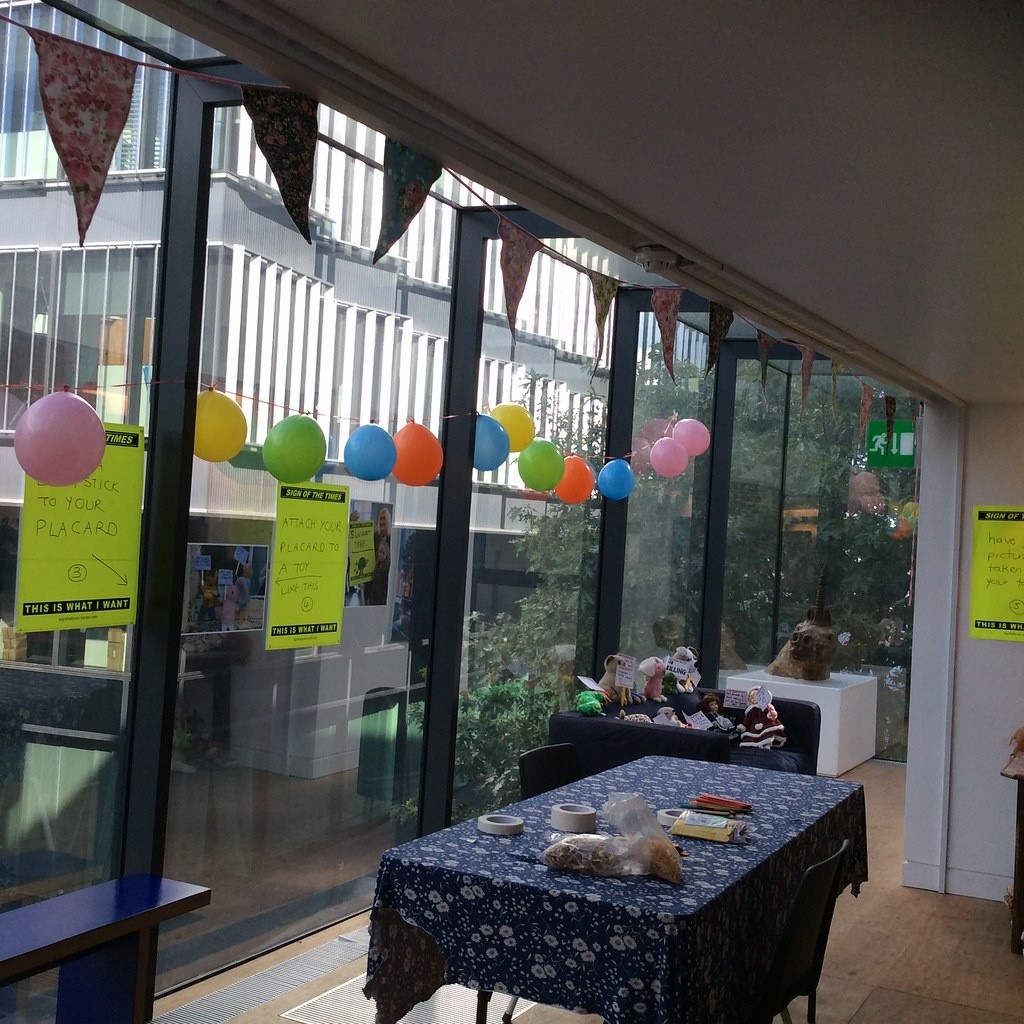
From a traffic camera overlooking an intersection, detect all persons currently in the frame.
[366,539,388,605]
[349,505,390,607]
[872,606,907,669]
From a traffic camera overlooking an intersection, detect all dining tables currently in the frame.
[382,755,864,1024]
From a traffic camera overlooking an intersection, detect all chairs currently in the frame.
[749,837,849,1024]
[518,742,576,801]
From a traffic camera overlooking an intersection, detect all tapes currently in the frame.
[476,814,524,834]
[656,808,686,825]
[551,804,597,833]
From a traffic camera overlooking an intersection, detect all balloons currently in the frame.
[598,457,637,502]
[901,515,919,538]
[889,516,911,540]
[846,469,862,513]
[14,383,108,493]
[384,418,444,489]
[888,499,904,517]
[673,417,712,459]
[898,499,920,518]
[551,452,595,504]
[472,412,511,471]
[640,416,676,450]
[343,419,398,482]
[857,490,884,516]
[192,385,252,464]
[648,435,690,476]
[852,470,879,495]
[261,408,328,488]
[882,513,900,533]
[488,403,535,452]
[631,435,654,478]
[517,435,567,493]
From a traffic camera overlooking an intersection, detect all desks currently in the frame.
[1001,752,1024,954]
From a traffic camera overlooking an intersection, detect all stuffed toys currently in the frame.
[631,689,651,702]
[700,691,740,749]
[658,704,687,728]
[233,562,255,618]
[596,655,631,705]
[735,684,787,751]
[615,710,653,724]
[572,687,613,717]
[661,643,701,696]
[660,670,687,697]
[636,655,668,704]
[196,573,219,624]
[221,585,242,634]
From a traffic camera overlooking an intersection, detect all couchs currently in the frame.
[547,686,822,776]
